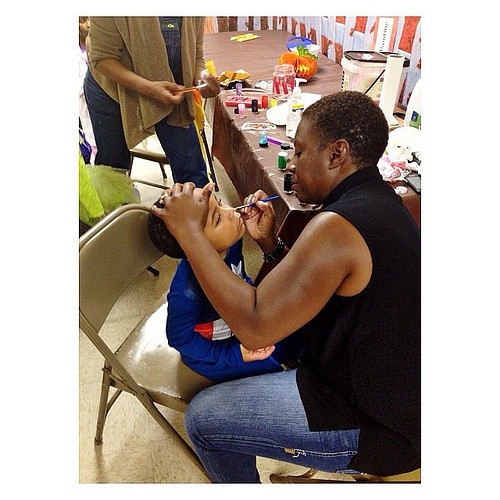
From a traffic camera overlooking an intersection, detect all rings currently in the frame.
[159,194,171,204]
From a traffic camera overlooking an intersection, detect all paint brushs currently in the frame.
[233,195,280,211]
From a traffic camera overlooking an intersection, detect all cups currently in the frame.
[252,100,258,112]
[261,95,278,110]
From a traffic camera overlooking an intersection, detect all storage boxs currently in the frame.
[340,50,410,119]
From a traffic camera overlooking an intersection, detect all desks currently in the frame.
[196,29,421,239]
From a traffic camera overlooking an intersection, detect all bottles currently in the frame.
[272,65,296,101]
[280,142,290,163]
[236,83,243,96]
[257,130,269,147]
[277,152,288,171]
[238,103,246,119]
[284,173,293,193]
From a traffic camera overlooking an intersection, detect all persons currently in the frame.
[82,16,220,190]
[79,16,99,165]
[152,91,421,484]
[147,188,301,382]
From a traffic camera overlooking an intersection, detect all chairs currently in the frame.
[79,133,421,484]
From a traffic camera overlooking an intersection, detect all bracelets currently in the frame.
[262,237,285,263]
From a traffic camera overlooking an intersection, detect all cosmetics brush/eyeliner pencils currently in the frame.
[236,195,280,209]
